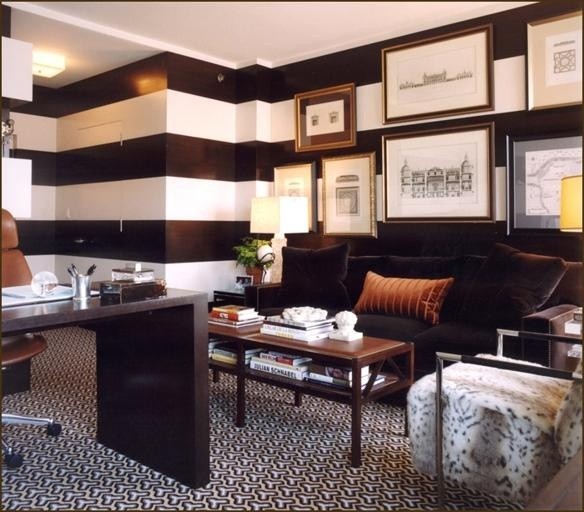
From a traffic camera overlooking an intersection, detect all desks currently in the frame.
[214,288,244,305]
[207,318,415,469]
[0,281,211,488]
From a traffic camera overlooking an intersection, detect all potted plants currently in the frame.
[233,237,275,285]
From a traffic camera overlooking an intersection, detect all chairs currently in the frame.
[1,207,61,469]
[407,328,584,511]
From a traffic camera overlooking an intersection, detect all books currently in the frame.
[207,339,262,370]
[263,349,315,381]
[208,304,266,328]
[315,364,386,390]
[260,316,338,343]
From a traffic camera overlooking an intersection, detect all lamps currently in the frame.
[32,50,66,79]
[560,175,584,320]
[250,196,309,283]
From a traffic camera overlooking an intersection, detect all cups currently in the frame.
[71,274,93,304]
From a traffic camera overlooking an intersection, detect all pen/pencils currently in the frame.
[86,264,96,275]
[68,268,73,276]
[71,263,79,276]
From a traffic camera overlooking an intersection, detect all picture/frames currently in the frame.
[321,152,379,240]
[380,121,496,224]
[524,8,583,112]
[381,24,495,124]
[273,161,318,235]
[505,126,583,238]
[294,82,357,154]
[234,276,254,293]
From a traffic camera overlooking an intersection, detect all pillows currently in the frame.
[282,242,568,328]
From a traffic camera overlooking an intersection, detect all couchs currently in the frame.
[244,254,584,409]
